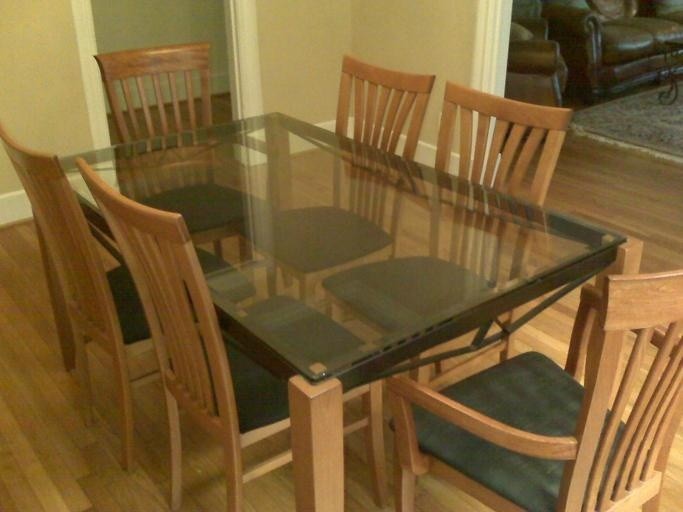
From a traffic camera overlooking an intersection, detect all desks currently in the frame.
[57,112,645,512]
[656,39,681,104]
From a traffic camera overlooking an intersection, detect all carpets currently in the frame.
[571,78,682,165]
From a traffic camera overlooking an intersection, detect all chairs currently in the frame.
[76,154,390,510]
[506,2,601,110]
[1,124,258,468]
[94,40,265,270]
[239,52,435,302]
[386,268,683,512]
[323,82,571,386]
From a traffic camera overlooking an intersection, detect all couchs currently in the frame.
[557,0,682,104]
